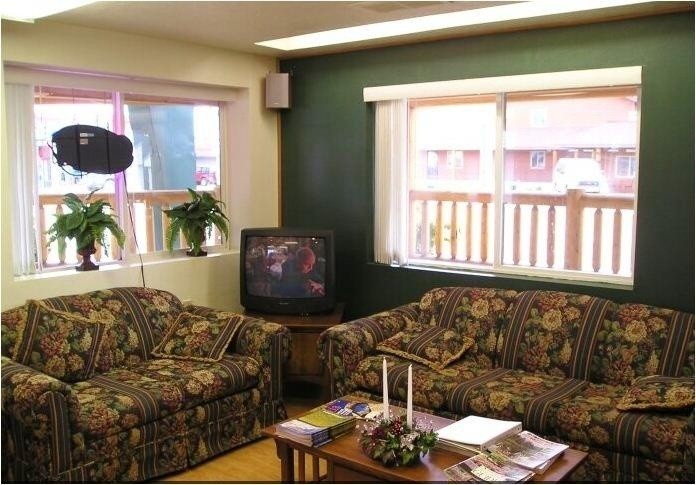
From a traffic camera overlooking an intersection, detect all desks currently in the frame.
[258,389,590,484]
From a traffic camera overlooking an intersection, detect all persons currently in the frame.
[258,243,325,298]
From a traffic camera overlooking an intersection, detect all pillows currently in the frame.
[375,315,478,371]
[616,374,694,415]
[9,297,109,384]
[149,310,244,362]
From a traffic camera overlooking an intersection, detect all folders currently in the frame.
[433,415,523,452]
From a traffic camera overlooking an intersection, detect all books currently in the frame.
[274,408,357,449]
[430,416,571,484]
[326,399,385,421]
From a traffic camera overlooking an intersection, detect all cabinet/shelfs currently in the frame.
[239,301,346,405]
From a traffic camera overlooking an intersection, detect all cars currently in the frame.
[613,175,637,193]
[551,157,610,196]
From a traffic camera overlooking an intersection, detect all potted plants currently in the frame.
[37,183,129,272]
[161,185,230,256]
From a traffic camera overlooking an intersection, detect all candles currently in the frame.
[381,356,389,422]
[405,364,413,429]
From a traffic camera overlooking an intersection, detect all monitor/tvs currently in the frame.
[236,224,343,315]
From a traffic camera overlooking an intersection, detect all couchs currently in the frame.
[2,285,295,484]
[315,285,694,484]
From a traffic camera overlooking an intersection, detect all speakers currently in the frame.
[263,70,292,111]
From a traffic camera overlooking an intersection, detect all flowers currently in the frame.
[355,408,439,467]
